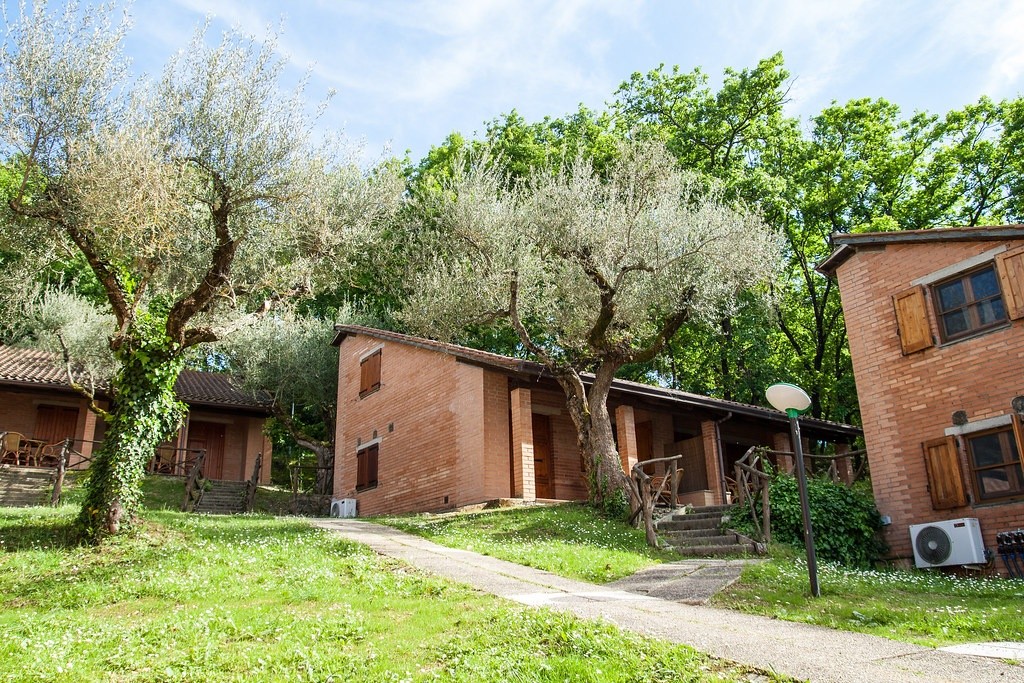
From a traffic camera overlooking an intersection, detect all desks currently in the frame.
[19,439,48,466]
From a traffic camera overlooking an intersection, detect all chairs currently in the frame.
[158,445,174,472]
[39,440,65,467]
[0,432,29,466]
[651,468,684,504]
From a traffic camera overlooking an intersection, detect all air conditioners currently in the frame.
[331,498,356,518]
[908,518,987,568]
